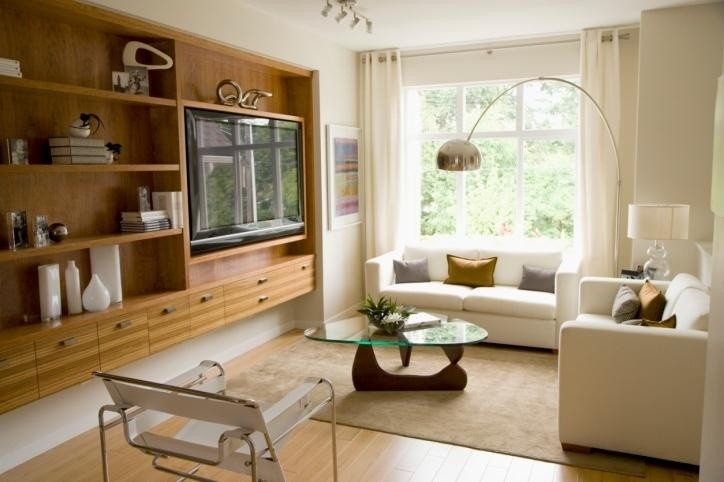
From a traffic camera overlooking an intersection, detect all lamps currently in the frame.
[92,358,341,481]
[627,203,691,279]
[321,0,376,35]
[435,76,623,276]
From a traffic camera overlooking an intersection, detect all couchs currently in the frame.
[364,246,583,353]
[557,271,712,468]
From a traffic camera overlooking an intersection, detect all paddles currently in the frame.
[212,335,649,480]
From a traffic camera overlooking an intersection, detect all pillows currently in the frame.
[443,253,499,288]
[392,256,433,285]
[612,281,676,327]
[517,264,558,295]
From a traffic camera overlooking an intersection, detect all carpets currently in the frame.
[212,335,649,480]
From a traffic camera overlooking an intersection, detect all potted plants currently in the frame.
[326,122,366,232]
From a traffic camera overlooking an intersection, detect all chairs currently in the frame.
[92,358,341,481]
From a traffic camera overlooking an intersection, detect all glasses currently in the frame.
[435,76,623,276]
[627,203,691,279]
[321,0,376,35]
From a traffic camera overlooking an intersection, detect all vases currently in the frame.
[368,321,403,335]
[358,289,419,337]
[36,243,122,324]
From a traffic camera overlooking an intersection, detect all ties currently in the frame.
[184,106,305,255]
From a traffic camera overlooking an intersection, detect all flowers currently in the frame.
[358,289,419,337]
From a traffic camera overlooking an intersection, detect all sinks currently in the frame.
[557,271,712,468]
[364,246,583,353]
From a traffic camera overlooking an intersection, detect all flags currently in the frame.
[36,243,122,324]
[368,321,403,335]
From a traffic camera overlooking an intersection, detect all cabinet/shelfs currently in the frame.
[64,260,82,315]
[0,0,319,415]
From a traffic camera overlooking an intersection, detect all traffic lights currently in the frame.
[517,264,558,295]
[392,256,433,285]
[612,281,676,327]
[443,253,499,288]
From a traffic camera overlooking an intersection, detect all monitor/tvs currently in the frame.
[52,155,108,165]
[0,57,21,66]
[48,136,103,147]
[0,64,20,71]
[49,145,106,157]
[117,192,183,232]
[0,69,23,79]
[400,311,443,329]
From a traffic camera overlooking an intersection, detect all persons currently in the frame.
[130,70,143,92]
[13,213,28,249]
[13,136,30,165]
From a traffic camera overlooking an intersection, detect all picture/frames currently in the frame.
[326,122,366,232]
